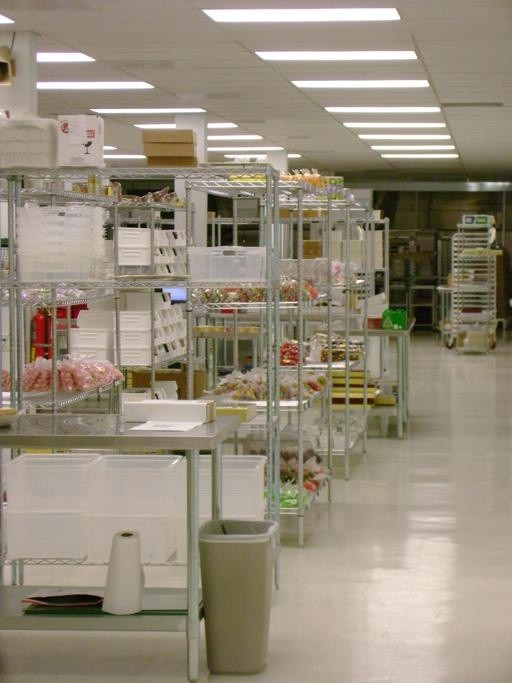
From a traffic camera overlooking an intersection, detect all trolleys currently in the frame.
[443,229,503,351]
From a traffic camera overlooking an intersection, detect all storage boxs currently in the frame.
[57,116,105,165]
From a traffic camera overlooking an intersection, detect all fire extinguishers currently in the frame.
[28,307,52,363]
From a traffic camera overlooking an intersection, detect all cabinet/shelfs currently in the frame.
[450,223,497,350]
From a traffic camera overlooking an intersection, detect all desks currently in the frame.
[0,410,237,683]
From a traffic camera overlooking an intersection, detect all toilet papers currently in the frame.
[101,527,146,616]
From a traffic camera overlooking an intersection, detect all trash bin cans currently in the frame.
[198,518,279,676]
